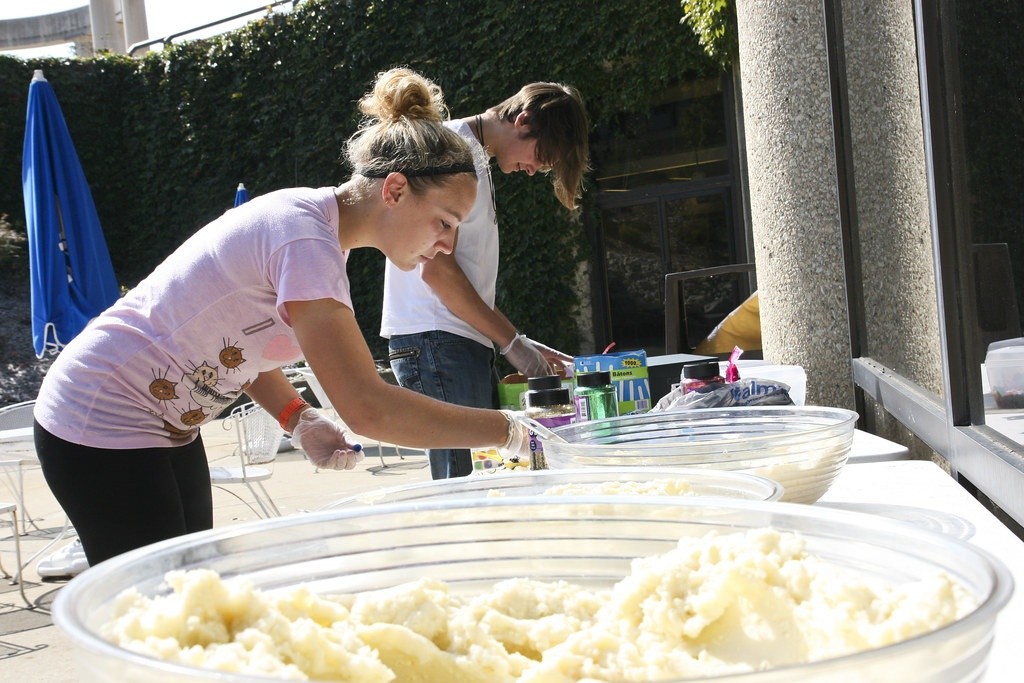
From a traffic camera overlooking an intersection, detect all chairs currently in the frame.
[0,502,42,608]
[207,386,308,519]
[295,365,407,468]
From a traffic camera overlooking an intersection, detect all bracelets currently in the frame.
[277,396,310,432]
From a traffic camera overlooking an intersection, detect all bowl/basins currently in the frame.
[54,406,1017,683]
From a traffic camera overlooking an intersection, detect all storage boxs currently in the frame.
[645,352,719,411]
[574,349,652,416]
[496,372,575,413]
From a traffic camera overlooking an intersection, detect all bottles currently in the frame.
[523,370,618,470]
[680,361,725,397]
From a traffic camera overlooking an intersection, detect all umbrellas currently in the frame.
[22,66,125,358]
[231,178,250,209]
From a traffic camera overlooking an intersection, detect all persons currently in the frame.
[27,63,542,570]
[379,79,594,483]
